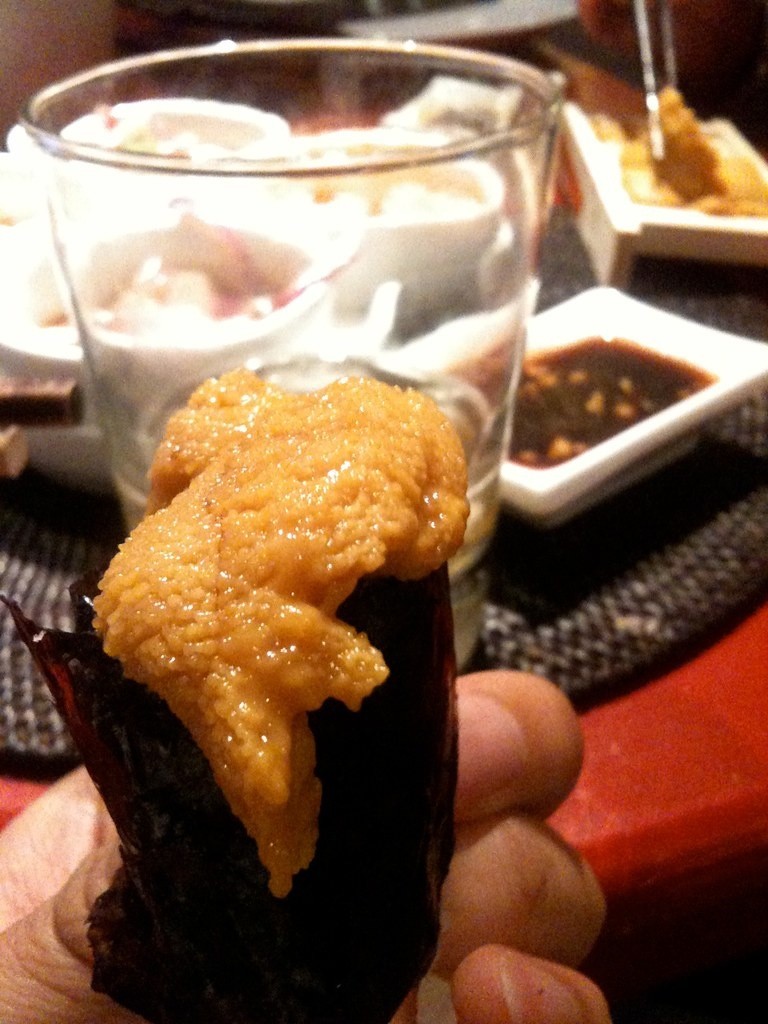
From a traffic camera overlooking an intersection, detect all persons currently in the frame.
[0,665,614,1024]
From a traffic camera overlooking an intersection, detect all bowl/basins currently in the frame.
[0,137,508,507]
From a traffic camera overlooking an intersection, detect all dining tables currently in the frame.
[4,104,765,896]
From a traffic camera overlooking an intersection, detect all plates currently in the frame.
[476,279,768,527]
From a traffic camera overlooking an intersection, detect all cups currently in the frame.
[25,27,559,686]
